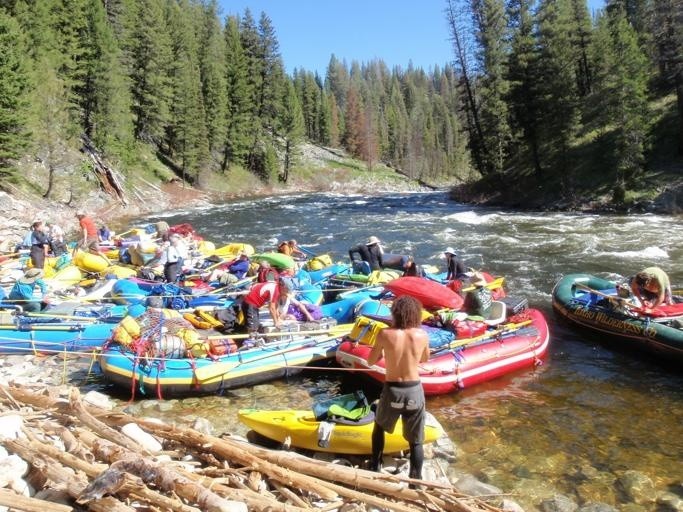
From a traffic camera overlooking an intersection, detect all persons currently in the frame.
[632,267,673,308]
[400,255,426,279]
[458,272,492,319]
[348,236,384,270]
[367,296,430,486]
[443,247,468,280]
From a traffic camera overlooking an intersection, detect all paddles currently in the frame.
[450,320,536,349]
[575,280,664,317]
[18,311,125,323]
[207,323,355,341]
[194,331,351,382]
[431,327,537,356]
[461,276,504,291]
[184,281,196,288]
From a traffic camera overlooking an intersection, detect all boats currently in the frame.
[235,407,443,456]
[551,272,682,360]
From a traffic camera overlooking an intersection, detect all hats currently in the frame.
[365,236,381,246]
[444,247,457,257]
